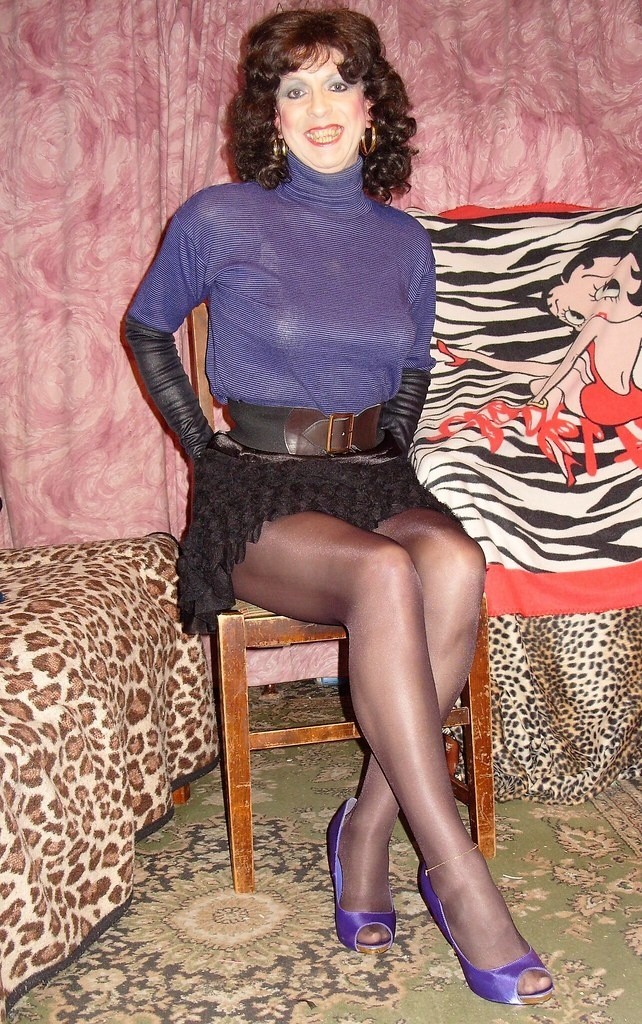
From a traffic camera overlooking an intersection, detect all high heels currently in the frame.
[326,797,396,955]
[418,863,553,1006]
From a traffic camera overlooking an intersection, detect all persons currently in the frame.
[124,7,554,1006]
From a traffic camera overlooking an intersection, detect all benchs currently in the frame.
[0,535,222,1024]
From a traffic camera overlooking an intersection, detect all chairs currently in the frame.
[188,299,495,893]
[402,204,642,804]
